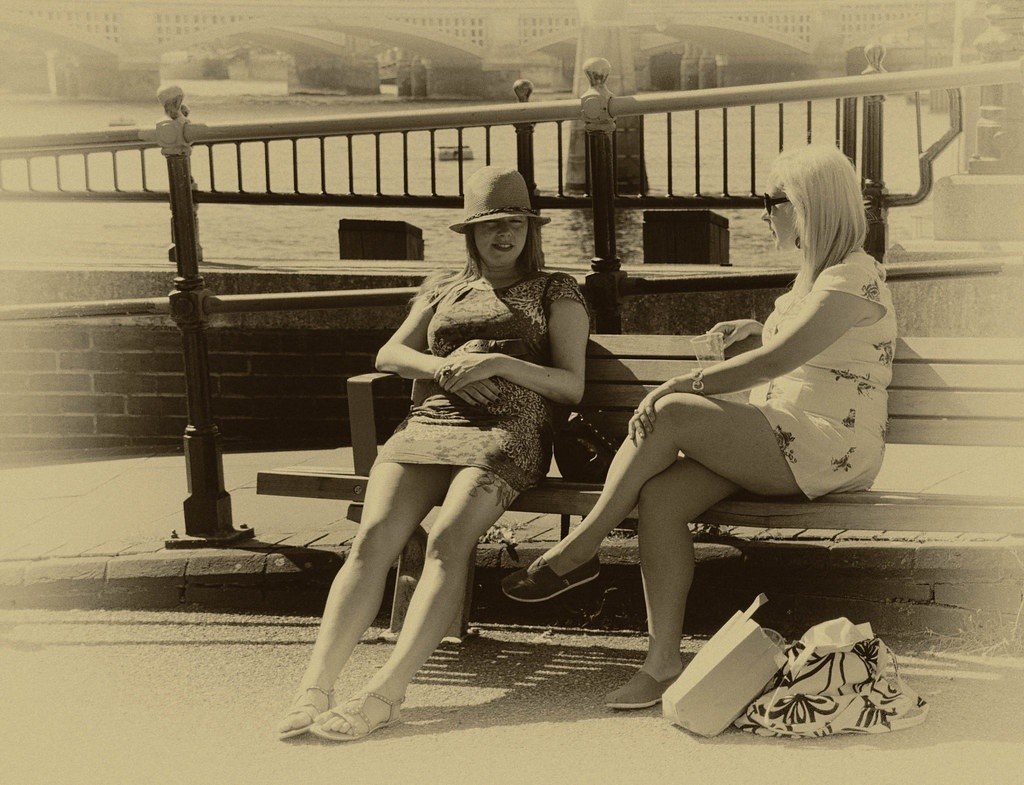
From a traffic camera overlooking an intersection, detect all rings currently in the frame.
[441,365,454,378]
[633,409,639,415]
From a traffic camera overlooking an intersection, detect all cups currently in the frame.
[690,332,724,368]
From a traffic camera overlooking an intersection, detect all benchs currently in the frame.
[257,334,1024,641]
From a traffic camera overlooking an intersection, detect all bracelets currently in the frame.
[691,367,706,396]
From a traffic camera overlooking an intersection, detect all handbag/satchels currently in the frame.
[732,617,928,739]
[562,408,620,482]
[661,593,789,738]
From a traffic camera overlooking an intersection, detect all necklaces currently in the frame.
[481,280,486,284]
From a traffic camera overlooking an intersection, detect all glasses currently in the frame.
[764,192,789,215]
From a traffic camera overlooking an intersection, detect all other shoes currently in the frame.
[501,553,600,602]
[603,670,684,709]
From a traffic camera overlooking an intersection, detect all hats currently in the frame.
[449,166,551,234]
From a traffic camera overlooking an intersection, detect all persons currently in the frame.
[276,166,592,740]
[500,142,897,709]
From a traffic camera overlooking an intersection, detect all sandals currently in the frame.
[310,692,406,742]
[279,685,336,739]
